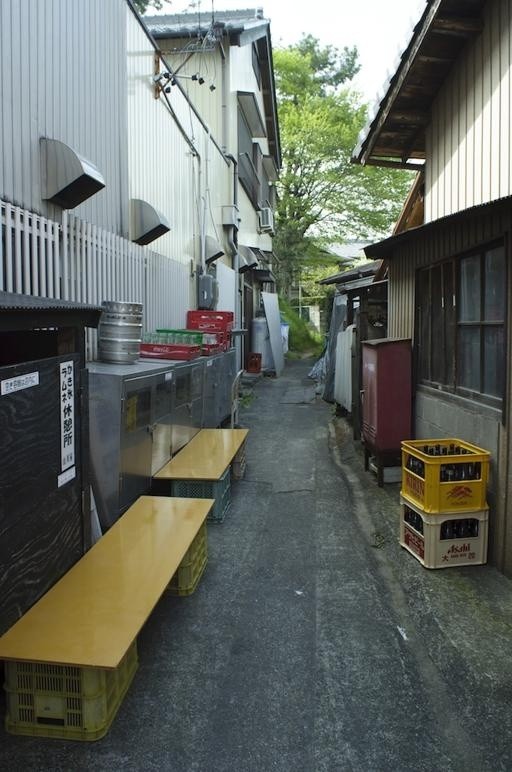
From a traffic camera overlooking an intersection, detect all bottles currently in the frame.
[140,331,199,348]
[403,503,479,540]
[406,443,484,482]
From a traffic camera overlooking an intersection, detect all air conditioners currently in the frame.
[260,206,274,228]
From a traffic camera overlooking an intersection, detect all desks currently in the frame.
[153,431,249,525]
[1,496,215,743]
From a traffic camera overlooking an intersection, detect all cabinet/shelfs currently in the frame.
[202,346,237,428]
[78,362,175,531]
[140,354,205,456]
